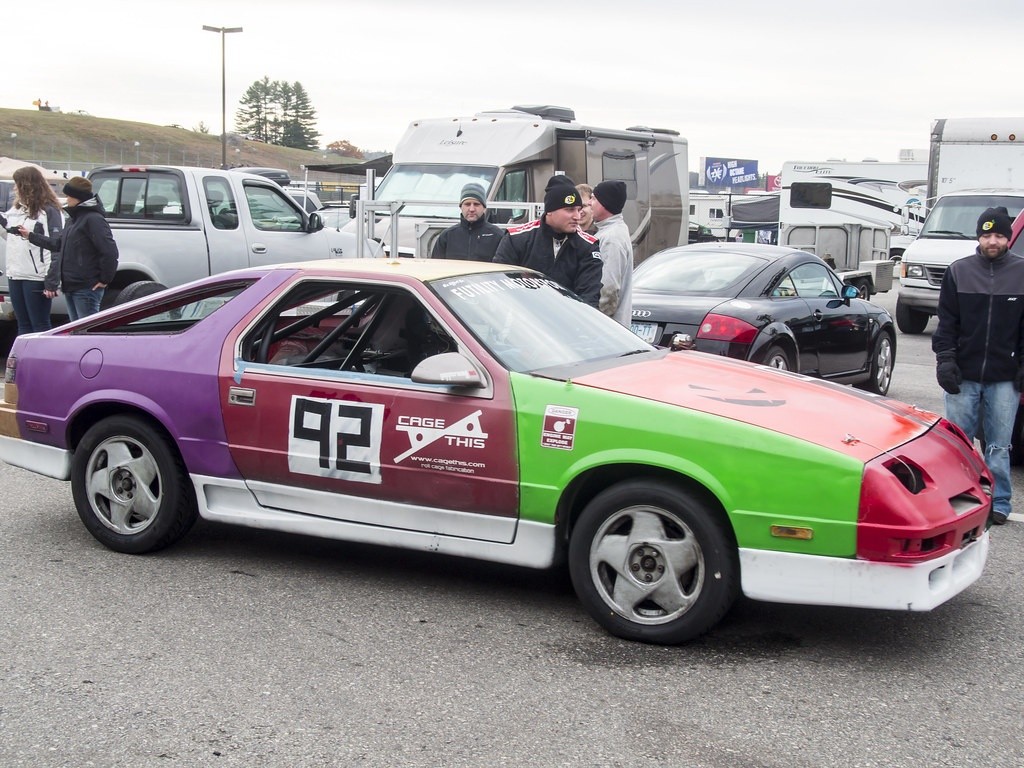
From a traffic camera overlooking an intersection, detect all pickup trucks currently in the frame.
[0,164,386,326]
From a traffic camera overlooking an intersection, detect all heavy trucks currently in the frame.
[777,158,928,261]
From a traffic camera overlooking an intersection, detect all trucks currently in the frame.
[688,189,742,239]
[339,105,689,264]
[894,116,1024,335]
[0,157,71,212]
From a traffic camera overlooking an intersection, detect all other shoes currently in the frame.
[991,512,1007,526]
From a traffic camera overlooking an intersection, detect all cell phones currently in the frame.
[11,226,21,234]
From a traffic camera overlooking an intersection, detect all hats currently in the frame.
[594,181,626,216]
[545,175,583,214]
[976,206,1013,240]
[63,177,93,200]
[459,182,486,207]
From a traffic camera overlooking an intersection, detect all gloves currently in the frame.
[936,351,962,395]
[1014,361,1024,394]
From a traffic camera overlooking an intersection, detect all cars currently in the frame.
[310,205,351,230]
[630,243,899,396]
[0,259,992,645]
[1008,210,1023,257]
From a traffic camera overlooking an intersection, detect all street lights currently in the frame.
[203,24,243,171]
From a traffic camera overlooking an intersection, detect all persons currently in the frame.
[491,175,604,311]
[429,182,504,263]
[935,206,1024,525]
[0,166,62,340]
[575,181,633,329]
[16,176,118,322]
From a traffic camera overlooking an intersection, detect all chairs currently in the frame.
[140,196,168,214]
[207,189,224,214]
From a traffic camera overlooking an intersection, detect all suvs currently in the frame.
[135,166,323,215]
